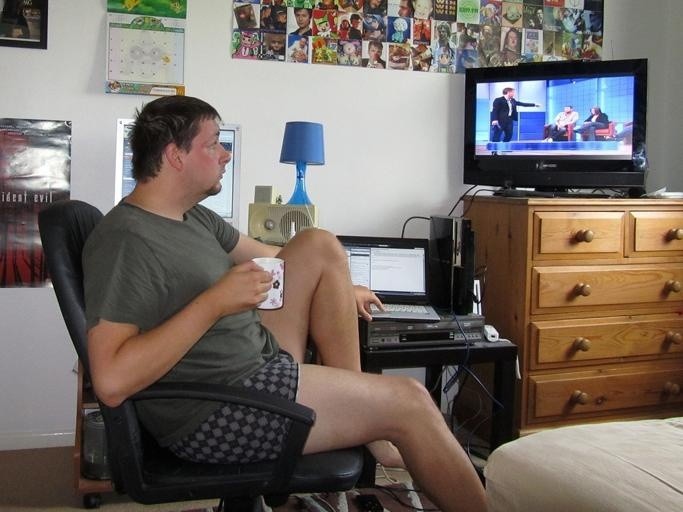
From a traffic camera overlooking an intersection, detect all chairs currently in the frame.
[39,200,364,512]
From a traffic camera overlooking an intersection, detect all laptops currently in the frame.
[336,236,441,321]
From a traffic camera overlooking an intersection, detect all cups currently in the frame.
[251,257,283,312]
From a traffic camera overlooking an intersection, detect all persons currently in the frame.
[260,0,543,72]
[543,106,580,143]
[573,106,609,141]
[613,127,632,141]
[81,95,488,512]
[489,87,540,156]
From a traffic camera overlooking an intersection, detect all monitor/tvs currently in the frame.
[114,119,241,230]
[464,58,648,199]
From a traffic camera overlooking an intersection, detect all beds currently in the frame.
[482,417,683,512]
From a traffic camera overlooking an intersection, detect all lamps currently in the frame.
[280,122,326,205]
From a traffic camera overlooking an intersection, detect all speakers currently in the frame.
[248,204,319,246]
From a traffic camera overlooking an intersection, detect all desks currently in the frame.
[359,329,518,487]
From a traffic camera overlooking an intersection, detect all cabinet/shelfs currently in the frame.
[461,194,683,440]
